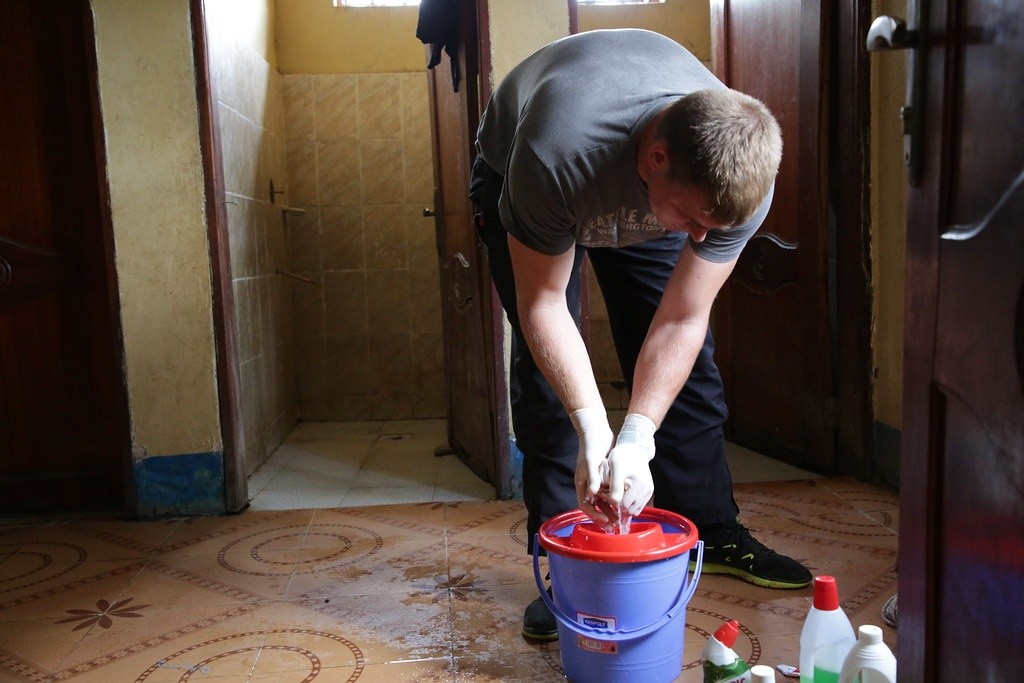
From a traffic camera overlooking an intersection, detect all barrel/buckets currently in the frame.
[533,507,704,683]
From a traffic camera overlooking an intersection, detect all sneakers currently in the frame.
[689,515,814,589]
[521,570,561,640]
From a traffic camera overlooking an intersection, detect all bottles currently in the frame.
[840,625,897,683]
[799,575,860,683]
[751,664,775,683]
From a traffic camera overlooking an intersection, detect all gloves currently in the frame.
[570,408,619,531]
[602,414,658,517]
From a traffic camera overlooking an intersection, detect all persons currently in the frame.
[469,27,815,641]
